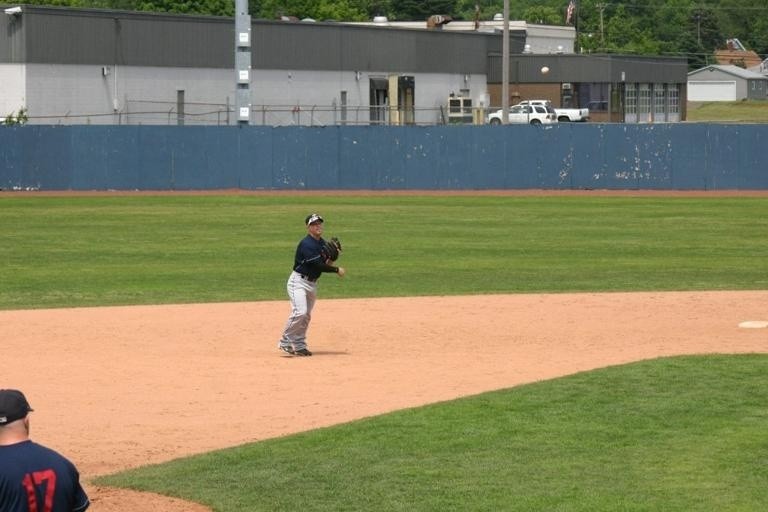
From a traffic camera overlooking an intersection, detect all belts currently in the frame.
[301,275,316,283]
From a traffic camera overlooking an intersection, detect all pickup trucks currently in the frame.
[519,99,590,122]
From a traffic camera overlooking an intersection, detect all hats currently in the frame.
[306,214,323,226]
[0,389,34,424]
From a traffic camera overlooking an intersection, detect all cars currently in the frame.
[486,104,558,126]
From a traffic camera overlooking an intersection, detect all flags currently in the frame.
[565,1,575,24]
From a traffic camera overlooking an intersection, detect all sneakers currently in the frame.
[278,340,312,356]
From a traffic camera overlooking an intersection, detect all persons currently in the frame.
[0,388,91,512]
[278,214,347,357]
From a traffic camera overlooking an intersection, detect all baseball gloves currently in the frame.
[321,237,341,261]
